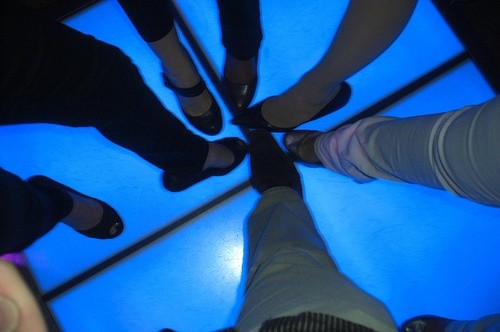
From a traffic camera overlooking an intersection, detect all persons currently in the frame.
[118,0,500,332]
[0,0,248,258]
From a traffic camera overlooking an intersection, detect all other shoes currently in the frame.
[27,174,124,240]
[284,130,323,165]
[246,130,303,198]
[163,86,222,136]
[162,137,246,192]
[231,86,351,132]
[214,86,257,114]
